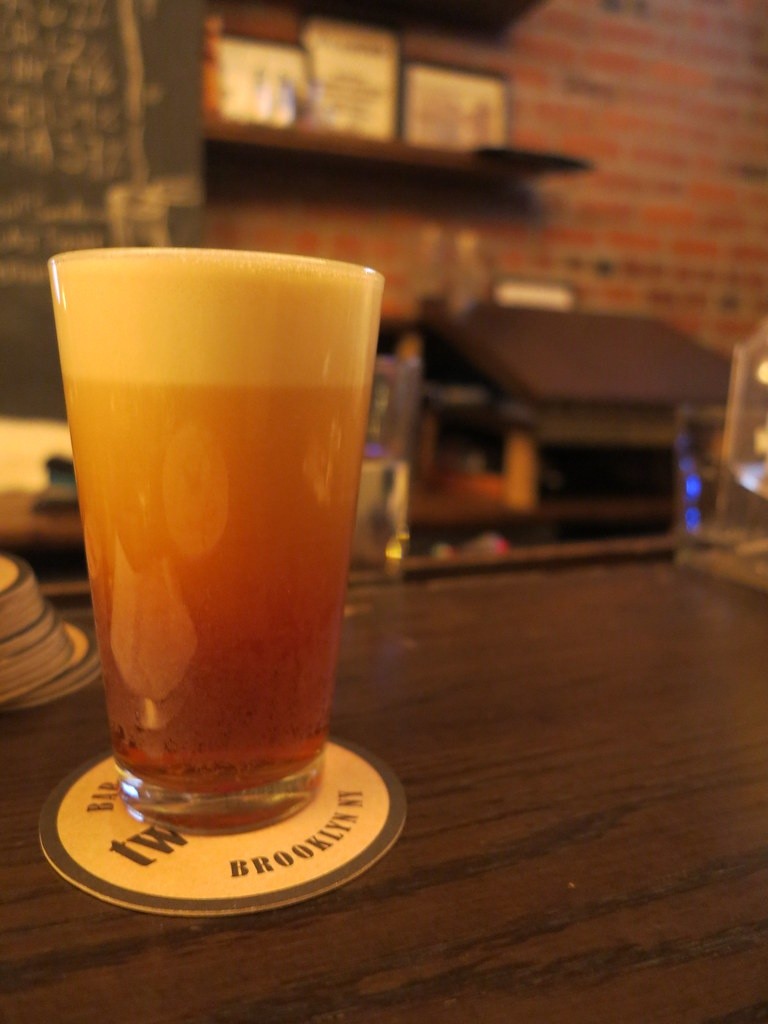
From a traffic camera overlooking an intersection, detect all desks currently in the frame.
[0,537,768,1024]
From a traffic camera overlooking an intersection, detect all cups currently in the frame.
[0,554,74,705]
[46,249,385,831]
[714,312,768,544]
[352,356,421,566]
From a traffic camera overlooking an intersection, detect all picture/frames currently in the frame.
[297,14,405,141]
[404,56,512,153]
[221,35,302,127]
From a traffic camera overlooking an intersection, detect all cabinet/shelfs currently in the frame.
[198,0,597,189]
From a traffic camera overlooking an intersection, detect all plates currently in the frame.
[0,621,102,710]
